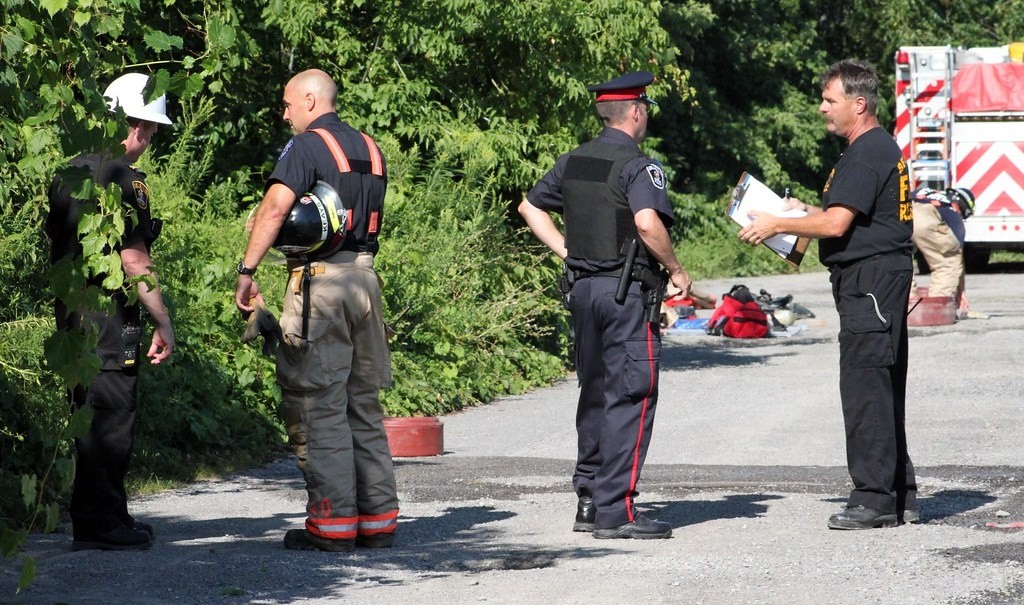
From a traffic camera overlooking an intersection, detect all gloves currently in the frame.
[239,298,284,356]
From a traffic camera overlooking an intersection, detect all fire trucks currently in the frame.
[893,44,1024,275]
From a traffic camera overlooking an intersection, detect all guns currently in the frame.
[630,263,669,325]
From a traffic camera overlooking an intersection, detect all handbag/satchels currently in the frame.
[664,285,716,309]
[704,285,767,338]
[750,287,816,318]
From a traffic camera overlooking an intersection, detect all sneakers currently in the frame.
[573,495,599,531]
[592,515,672,539]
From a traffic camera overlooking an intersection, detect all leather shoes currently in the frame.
[899,509,920,523]
[72,515,153,551]
[283,529,356,551]
[827,505,898,530]
[355,532,395,548]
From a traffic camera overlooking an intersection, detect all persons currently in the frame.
[738,58,920,531]
[911,187,975,317]
[236,69,399,552]
[519,75,692,540]
[47,72,176,550]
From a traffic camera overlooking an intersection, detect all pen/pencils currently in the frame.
[785,187,790,202]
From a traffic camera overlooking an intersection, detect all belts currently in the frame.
[574,265,623,278]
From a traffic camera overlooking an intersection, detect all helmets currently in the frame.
[245,179,348,265]
[101,73,173,125]
[946,187,976,219]
[764,305,795,330]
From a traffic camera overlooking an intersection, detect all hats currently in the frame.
[587,71,658,104]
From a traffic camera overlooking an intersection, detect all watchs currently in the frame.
[237,262,257,275]
